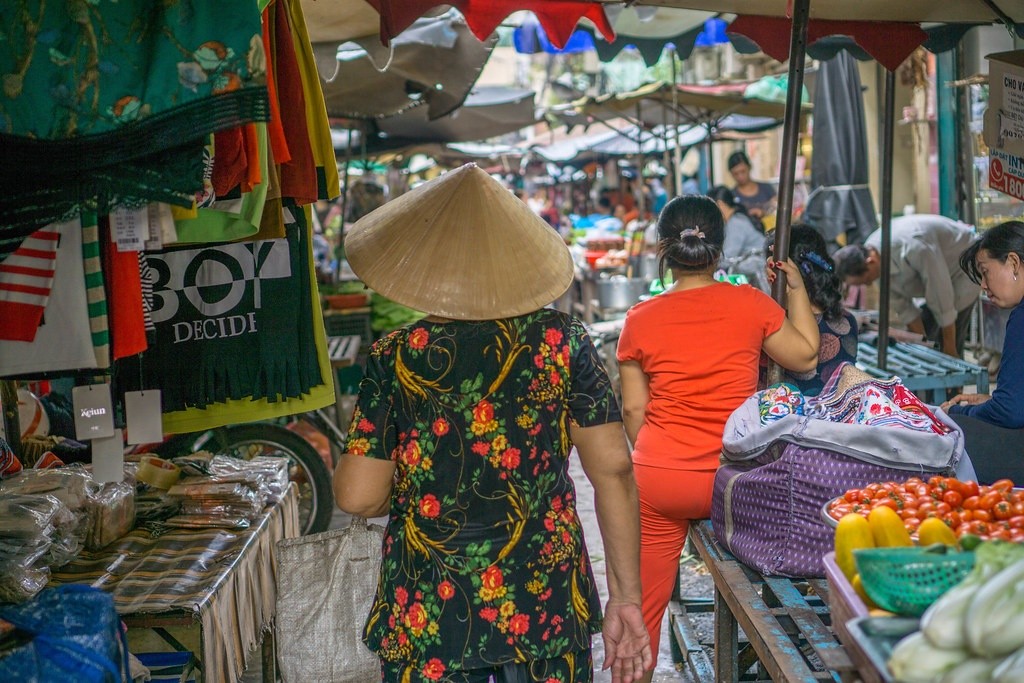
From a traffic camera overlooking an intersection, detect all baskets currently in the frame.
[853,547,977,618]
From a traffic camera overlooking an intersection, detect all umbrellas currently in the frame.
[804,49,879,256]
[314,7,810,285]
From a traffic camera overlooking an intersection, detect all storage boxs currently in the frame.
[822,552,898,683]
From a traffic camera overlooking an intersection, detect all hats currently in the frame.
[343,163,575,321]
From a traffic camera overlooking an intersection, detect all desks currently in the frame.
[325,335,362,472]
[0,455,300,683]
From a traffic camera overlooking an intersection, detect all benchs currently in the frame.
[669,519,810,662]
[686,560,877,683]
[855,342,991,405]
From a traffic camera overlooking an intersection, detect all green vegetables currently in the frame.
[887,540,1024,683]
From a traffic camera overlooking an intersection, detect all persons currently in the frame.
[617,194,820,683]
[765,222,858,397]
[705,187,771,259]
[939,221,1024,429]
[332,160,653,683]
[728,151,778,219]
[830,213,983,404]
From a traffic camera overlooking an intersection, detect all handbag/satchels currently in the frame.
[711,362,978,578]
[274,514,386,683]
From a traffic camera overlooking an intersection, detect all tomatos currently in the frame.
[828,475,1024,545]
